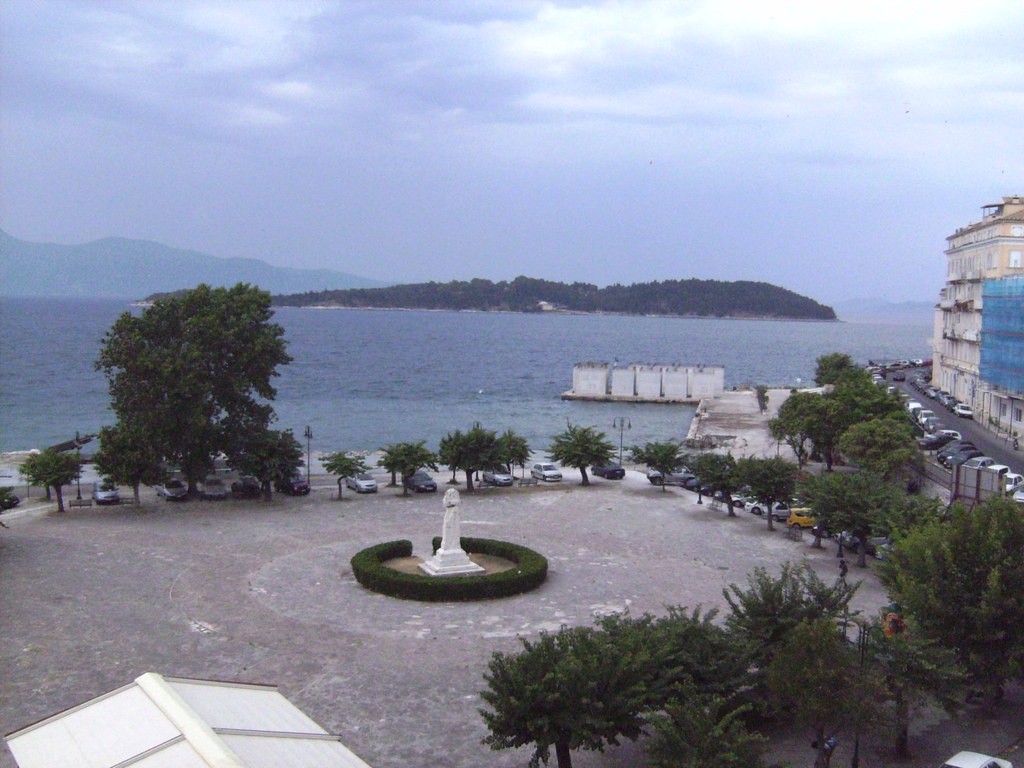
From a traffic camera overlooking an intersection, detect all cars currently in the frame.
[202,475,227,500]
[939,751,1013,768]
[273,474,311,497]
[646,465,895,561]
[591,461,626,481]
[530,463,563,482]
[91,480,121,506]
[482,467,514,487]
[232,475,262,498]
[864,358,1024,506]
[156,479,189,501]
[0,492,20,510]
[407,471,437,494]
[345,472,378,494]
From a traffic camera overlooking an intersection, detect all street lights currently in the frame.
[612,415,631,466]
[839,619,892,768]
[304,424,313,485]
